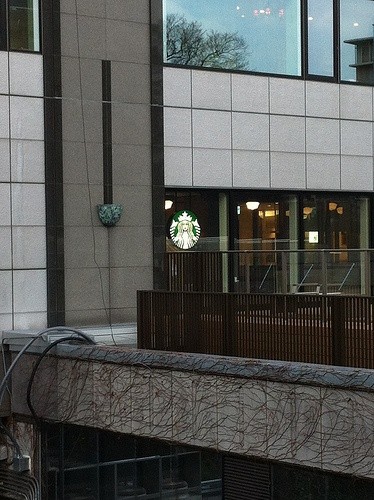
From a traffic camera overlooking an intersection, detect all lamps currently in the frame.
[328,203,337,210]
[303,207,314,214]
[246,202,261,210]
